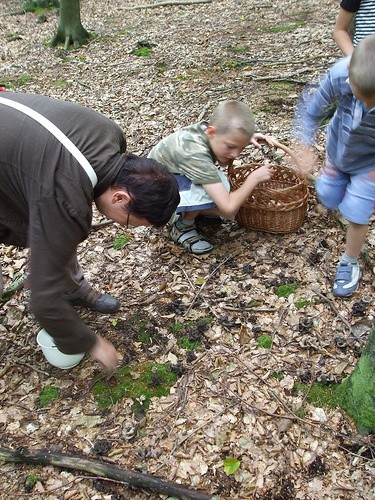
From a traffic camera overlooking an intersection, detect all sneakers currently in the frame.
[335,259,360,296]
[66,287,120,313]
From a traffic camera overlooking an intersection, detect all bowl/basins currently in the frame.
[36,327,85,370]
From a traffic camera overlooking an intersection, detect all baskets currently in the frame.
[231,138,309,233]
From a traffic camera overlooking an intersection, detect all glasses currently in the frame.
[126,210,135,229]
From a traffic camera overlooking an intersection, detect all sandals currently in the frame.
[169,220,214,253]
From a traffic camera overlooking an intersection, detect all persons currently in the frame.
[299,35,375,298]
[333,0,375,55]
[146,100,278,253]
[0,87,227,370]
